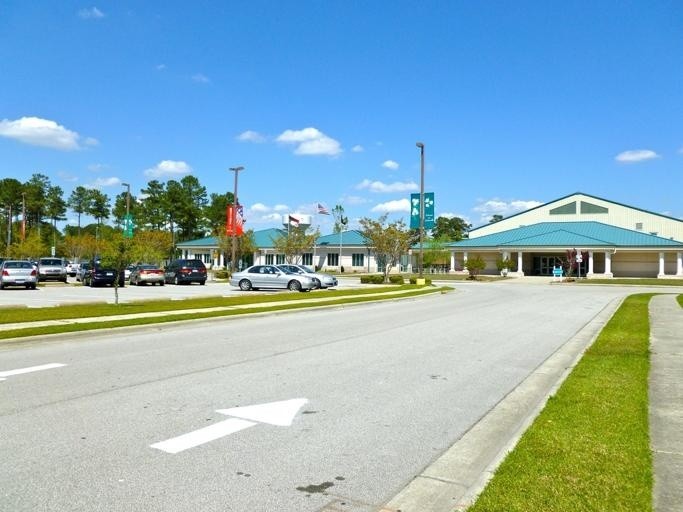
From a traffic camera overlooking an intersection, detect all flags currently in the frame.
[290,217,299,223]
[318,203,331,215]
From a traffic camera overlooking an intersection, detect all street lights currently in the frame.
[121,182,130,247]
[416,142,425,279]
[229,167,245,272]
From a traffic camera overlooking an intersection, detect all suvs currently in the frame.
[163,259,208,285]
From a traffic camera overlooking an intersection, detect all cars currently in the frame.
[129,265,165,287]
[0,256,131,290]
[229,263,339,291]
